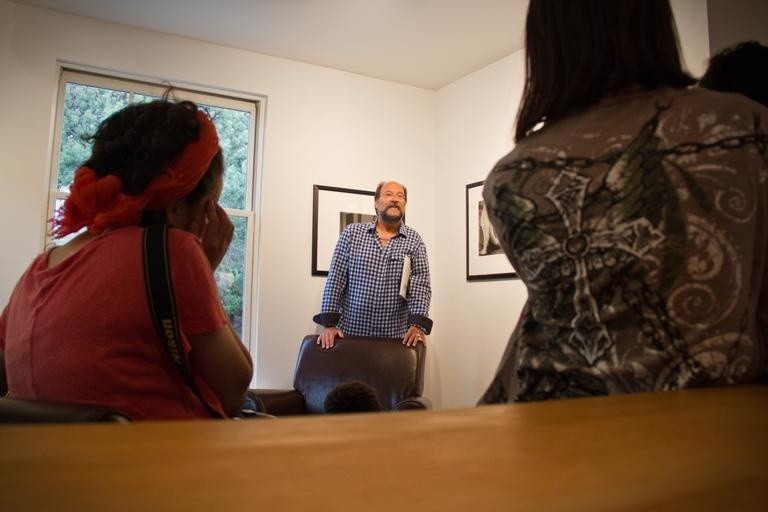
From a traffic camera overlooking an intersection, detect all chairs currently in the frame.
[293,336,425,411]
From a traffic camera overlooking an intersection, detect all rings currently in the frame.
[416,332,420,337]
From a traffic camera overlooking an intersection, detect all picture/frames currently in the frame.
[311,184,377,276]
[466,181,525,280]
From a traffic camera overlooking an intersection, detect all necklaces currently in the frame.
[379,235,390,242]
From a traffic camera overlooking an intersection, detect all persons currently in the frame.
[0,92,253,429]
[695,35,768,105]
[312,178,433,350]
[475,1,768,405]
